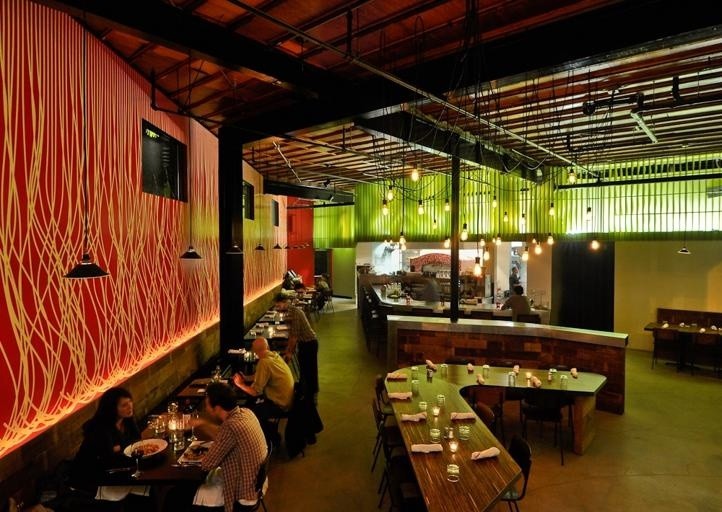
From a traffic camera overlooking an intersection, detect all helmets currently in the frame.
[122,437,169,459]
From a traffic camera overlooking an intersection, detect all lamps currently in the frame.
[96,348,300,512]
[369,355,608,511]
[359,284,384,356]
[409,307,542,322]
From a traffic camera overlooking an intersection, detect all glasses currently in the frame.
[179,43,205,261]
[225,68,244,255]
[254,135,314,251]
[676,142,692,256]
[380,163,603,278]
[62,7,113,281]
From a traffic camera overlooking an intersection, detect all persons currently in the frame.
[281,274,295,290]
[273,292,319,407]
[509,266,520,292]
[234,337,294,440]
[77,388,153,481]
[315,273,330,310]
[501,285,531,321]
[161,384,268,512]
[0,462,56,512]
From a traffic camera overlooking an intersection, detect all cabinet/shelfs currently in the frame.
[380,282,402,300]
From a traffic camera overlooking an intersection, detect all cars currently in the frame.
[481,364,570,387]
[410,360,470,483]
[151,400,198,454]
